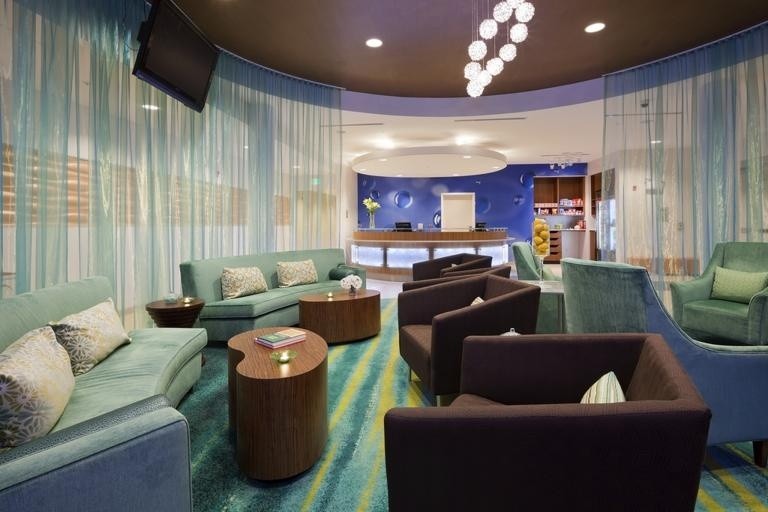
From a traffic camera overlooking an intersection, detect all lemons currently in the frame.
[533,220,550,256]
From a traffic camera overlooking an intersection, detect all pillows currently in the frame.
[711,266,768,304]
[579,371,626,404]
[47,298,131,377]
[220,267,268,299]
[277,258,318,287]
[0,326,74,448]
[452,263,456,267]
[470,296,483,305]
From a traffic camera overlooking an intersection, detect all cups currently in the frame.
[551,209,557,215]
[469,226,472,231]
[560,208,582,215]
[428,224,433,232]
[560,198,583,206]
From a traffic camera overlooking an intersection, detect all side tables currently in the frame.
[518,280,564,334]
[145,298,205,367]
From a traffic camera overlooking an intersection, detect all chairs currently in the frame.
[512,241,562,280]
[670,242,768,346]
[560,257,768,467]
[398,274,541,409]
[403,264,511,292]
[413,253,493,280]
[384,335,712,512]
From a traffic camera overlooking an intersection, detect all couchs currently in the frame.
[0,276,207,511]
[179,248,366,351]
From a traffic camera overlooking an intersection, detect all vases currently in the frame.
[369,212,375,229]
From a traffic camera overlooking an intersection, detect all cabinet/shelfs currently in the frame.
[544,231,560,262]
[533,177,584,215]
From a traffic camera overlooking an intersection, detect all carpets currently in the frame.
[175,298,768,512]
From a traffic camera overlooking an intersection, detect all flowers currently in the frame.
[363,198,381,211]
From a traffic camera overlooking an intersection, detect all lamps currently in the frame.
[464,0,535,98]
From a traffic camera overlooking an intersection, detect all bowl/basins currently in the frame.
[574,226,580,229]
[555,224,564,229]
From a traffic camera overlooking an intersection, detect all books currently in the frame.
[254,328,306,349]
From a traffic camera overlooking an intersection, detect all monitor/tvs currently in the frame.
[133,0,221,113]
[395,222,412,232]
[475,222,487,232]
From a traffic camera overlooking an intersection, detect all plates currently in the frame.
[270,350,297,363]
